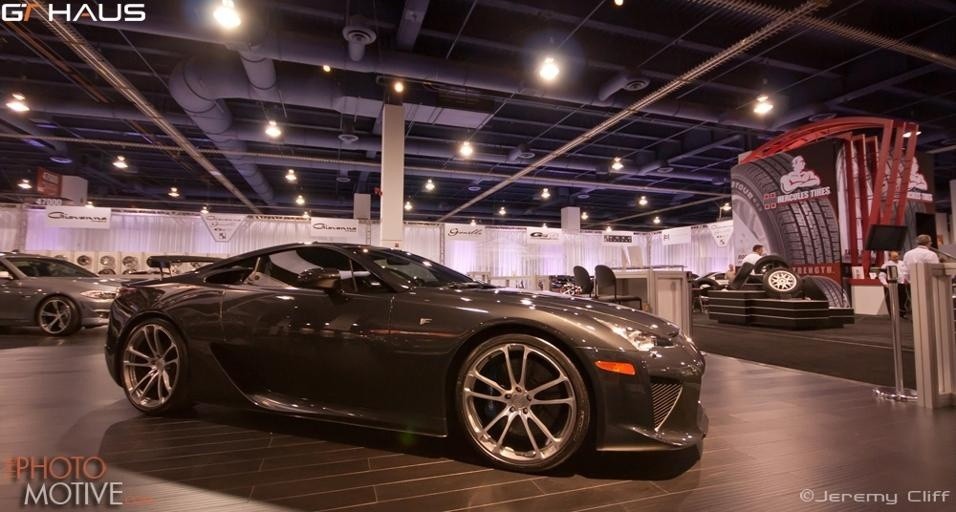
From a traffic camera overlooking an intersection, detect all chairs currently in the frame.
[593,264,642,311]
[571,265,601,299]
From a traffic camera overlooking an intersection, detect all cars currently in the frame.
[106,243,708,475]
[690,271,728,303]
[2,254,263,337]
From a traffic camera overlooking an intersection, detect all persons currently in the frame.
[878,251,908,321]
[742,245,767,274]
[903,234,940,291]
[723,264,735,280]
